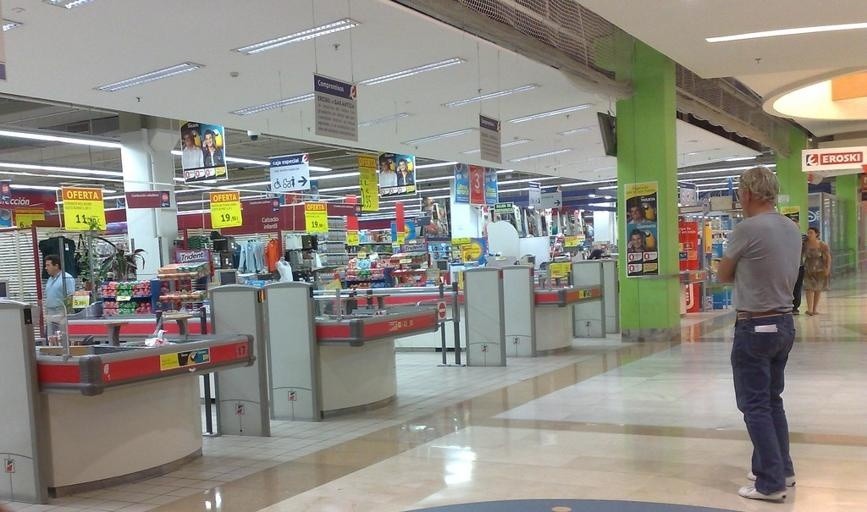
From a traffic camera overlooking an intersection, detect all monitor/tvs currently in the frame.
[0,279,10,300]
[214,269,238,285]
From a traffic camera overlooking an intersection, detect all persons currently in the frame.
[627,201,655,223]
[202,129,223,168]
[791,217,809,316]
[182,129,204,169]
[379,159,397,188]
[802,228,831,316]
[396,158,413,187]
[713,166,804,502]
[628,229,651,253]
[274,257,293,284]
[43,254,76,337]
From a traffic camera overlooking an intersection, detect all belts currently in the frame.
[736,310,775,320]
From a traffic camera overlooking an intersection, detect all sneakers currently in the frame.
[746,471,795,487]
[738,486,786,500]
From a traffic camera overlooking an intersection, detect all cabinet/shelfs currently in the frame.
[317,216,391,270]
